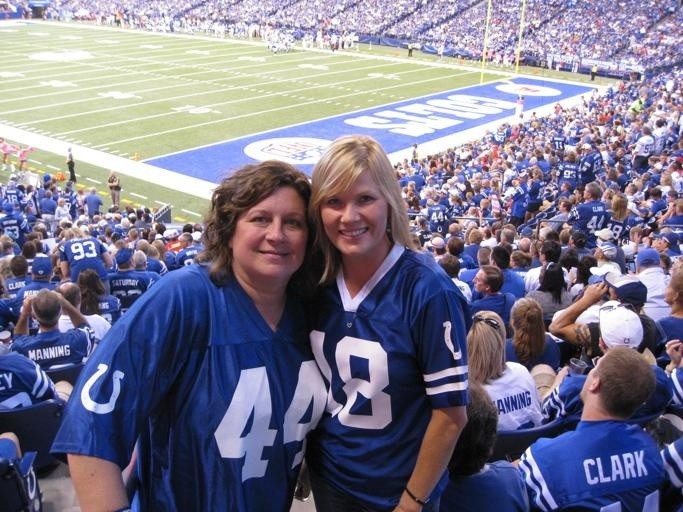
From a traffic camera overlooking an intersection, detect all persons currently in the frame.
[0,0,683,512]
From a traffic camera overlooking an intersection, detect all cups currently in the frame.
[569,356,587,377]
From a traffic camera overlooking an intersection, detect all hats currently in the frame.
[600,300,643,349]
[33,257,53,275]
[191,232,202,241]
[4,204,13,213]
[590,228,679,304]
[581,143,591,149]
[116,248,131,265]
[428,237,445,249]
[448,182,466,197]
[518,225,533,236]
[43,175,50,182]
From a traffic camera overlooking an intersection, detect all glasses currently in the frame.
[599,303,637,333]
[474,315,501,333]
